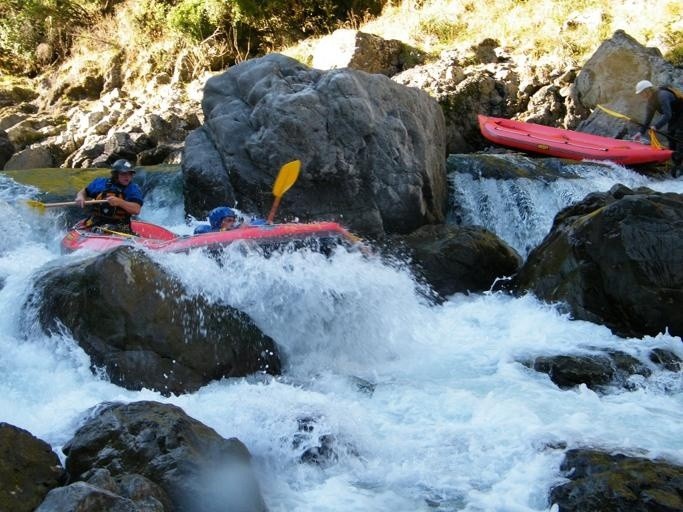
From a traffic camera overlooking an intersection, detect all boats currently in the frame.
[474,112,675,164]
[57,212,359,256]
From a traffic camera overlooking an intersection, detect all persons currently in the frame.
[631,80,682,179]
[193,206,269,236]
[72,159,144,239]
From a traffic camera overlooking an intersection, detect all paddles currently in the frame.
[22,196,110,214]
[596,103,669,137]
[265,158,302,224]
[96,225,170,245]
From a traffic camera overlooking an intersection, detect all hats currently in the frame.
[209,206,236,225]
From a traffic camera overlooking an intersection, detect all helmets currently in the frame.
[635,79,654,95]
[112,159,136,174]
